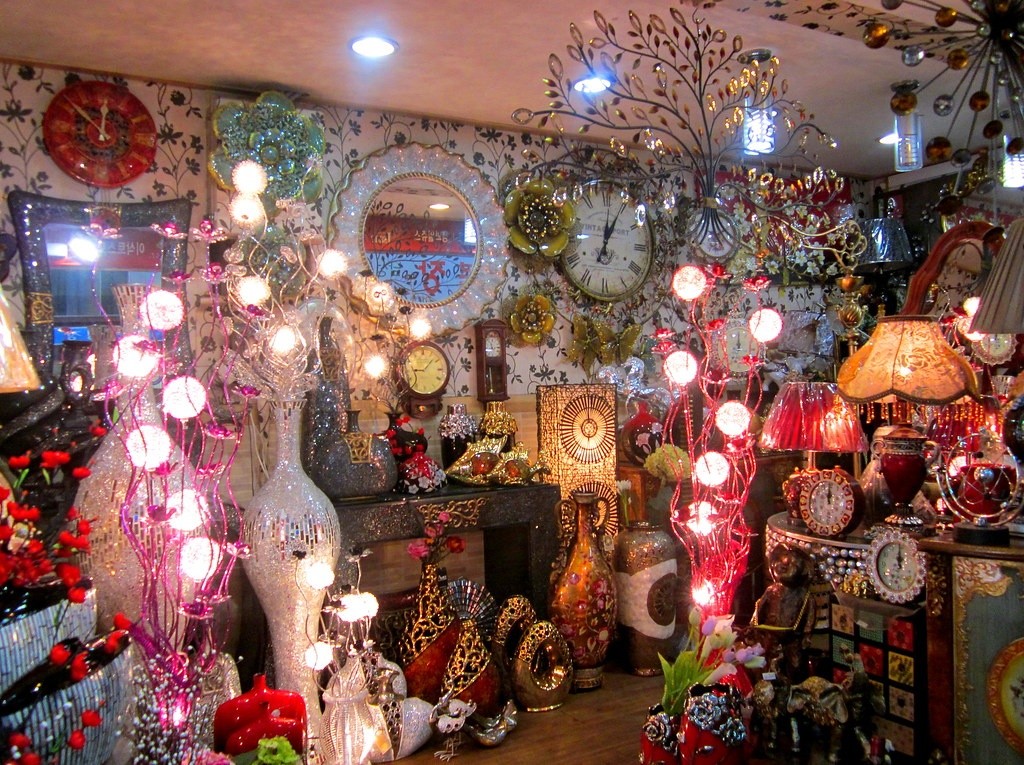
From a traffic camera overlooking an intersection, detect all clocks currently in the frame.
[554,177,656,304]
[474,317,511,402]
[395,342,452,418]
[43,81,157,187]
[868,528,927,604]
[687,210,740,264]
[800,469,864,539]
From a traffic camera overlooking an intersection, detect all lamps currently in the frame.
[738,47,777,153]
[101,165,390,764]
[891,78,923,172]
[998,110,1024,187]
[537,383,618,555]
[659,263,1024,667]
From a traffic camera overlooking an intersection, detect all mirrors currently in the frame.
[325,140,511,338]
[8,188,194,397]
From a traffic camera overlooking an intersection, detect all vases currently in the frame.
[549,493,617,691]
[439,402,476,470]
[312,409,396,503]
[69,282,211,763]
[395,560,500,718]
[612,520,678,674]
[237,398,342,764]
[396,443,448,497]
[479,400,519,453]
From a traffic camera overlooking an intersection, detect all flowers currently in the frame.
[657,605,766,714]
[406,510,464,561]
[385,414,425,454]
[0,420,131,764]
